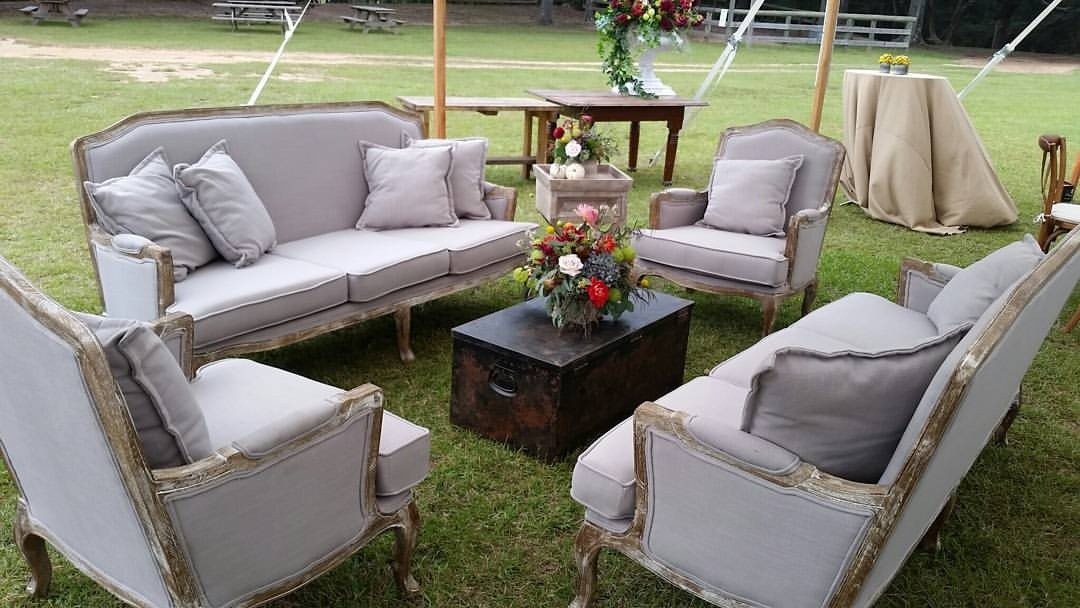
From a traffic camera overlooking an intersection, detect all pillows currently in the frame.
[702,155,1049,479]
[83,139,490,466]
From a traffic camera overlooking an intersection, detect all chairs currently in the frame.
[1037,133,1080,332]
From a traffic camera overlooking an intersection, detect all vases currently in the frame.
[569,161,598,177]
[880,63,890,73]
[611,34,681,97]
[894,65,908,75]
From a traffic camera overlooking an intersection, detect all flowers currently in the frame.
[592,0,708,100]
[513,115,657,341]
[880,53,911,65]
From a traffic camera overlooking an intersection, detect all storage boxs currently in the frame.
[450,285,695,464]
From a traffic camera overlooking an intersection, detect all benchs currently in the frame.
[69,101,539,372]
[568,224,1080,608]
[17,6,89,20]
[631,117,846,340]
[0,255,432,608]
[211,12,407,28]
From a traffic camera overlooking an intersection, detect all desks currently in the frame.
[212,0,399,34]
[32,0,81,26]
[398,97,684,188]
